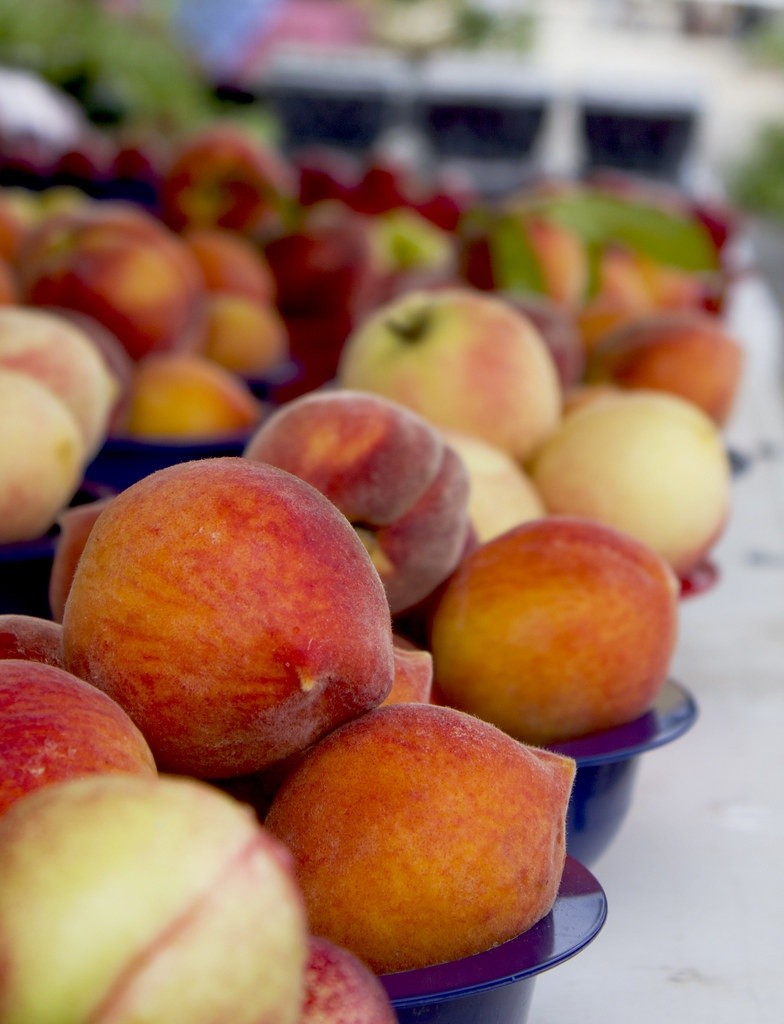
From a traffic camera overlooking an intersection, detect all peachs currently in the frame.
[0,123,753,1024]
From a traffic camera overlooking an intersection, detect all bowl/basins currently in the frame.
[0,481,117,617]
[83,438,247,493]
[247,362,300,401]
[375,854,607,1024]
[539,674,697,867]
